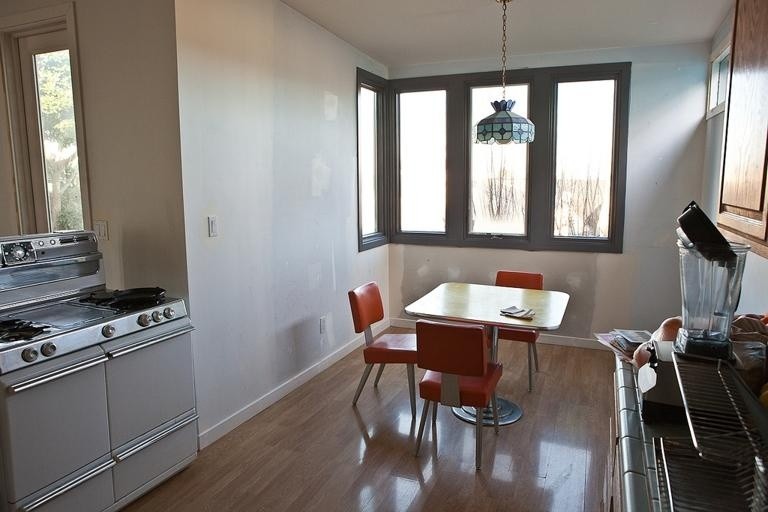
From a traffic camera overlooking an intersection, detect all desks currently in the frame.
[400,279,572,426]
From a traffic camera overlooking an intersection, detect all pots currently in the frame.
[90,288,166,303]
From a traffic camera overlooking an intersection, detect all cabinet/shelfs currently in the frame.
[101,319,200,512]
[713,0,767,262]
[597,369,626,512]
[0,347,113,512]
[653,352,767,512]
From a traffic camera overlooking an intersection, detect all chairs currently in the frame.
[480,270,543,396]
[407,316,507,474]
[348,281,432,419]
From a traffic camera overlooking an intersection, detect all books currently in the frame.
[609,325,653,358]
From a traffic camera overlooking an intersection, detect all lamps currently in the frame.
[469,0,536,148]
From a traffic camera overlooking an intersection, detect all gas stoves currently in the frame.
[0,286,188,376]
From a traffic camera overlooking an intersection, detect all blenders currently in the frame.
[671,200,753,361]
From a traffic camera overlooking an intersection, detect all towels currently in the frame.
[499,305,537,322]
